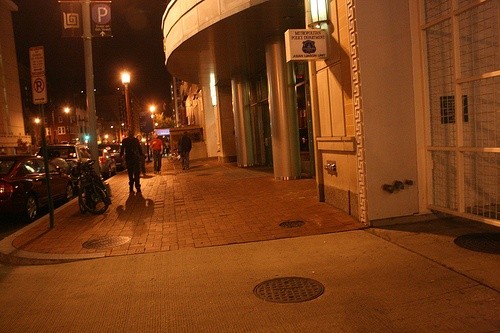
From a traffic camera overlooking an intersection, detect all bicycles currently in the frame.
[71,158,110,215]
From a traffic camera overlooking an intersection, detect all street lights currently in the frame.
[35,117,41,147]
[64,107,71,144]
[120,69,133,129]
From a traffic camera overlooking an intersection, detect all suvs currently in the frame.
[36,143,92,188]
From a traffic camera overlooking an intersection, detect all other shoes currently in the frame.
[130,188,133,192]
[137,188,140,191]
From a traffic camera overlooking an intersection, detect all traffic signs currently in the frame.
[29,45,45,77]
[91,1,114,38]
[32,75,47,105]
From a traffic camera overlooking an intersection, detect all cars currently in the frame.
[0,154,75,223]
[97,142,126,179]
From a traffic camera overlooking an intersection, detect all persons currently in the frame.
[177,132,192,170]
[120,128,146,191]
[148,131,164,176]
